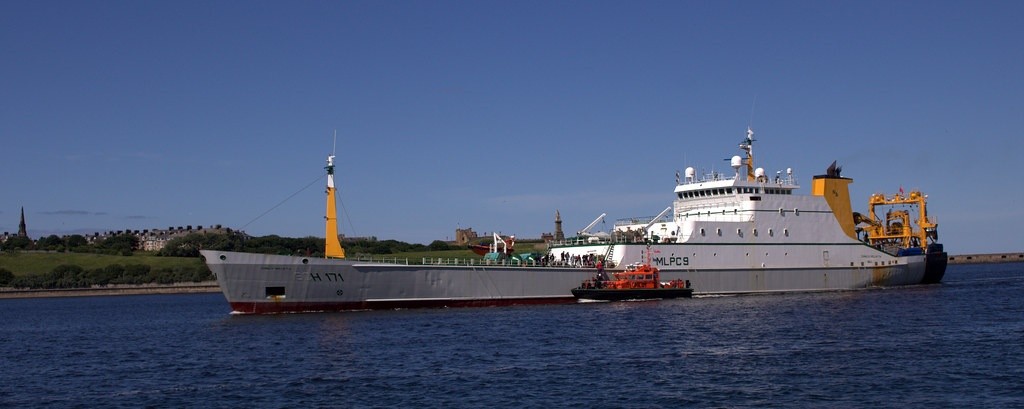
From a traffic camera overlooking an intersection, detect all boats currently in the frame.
[201,126,949,317]
[571,243,694,303]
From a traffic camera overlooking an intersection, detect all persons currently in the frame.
[542,249,616,289]
[622,226,670,243]
